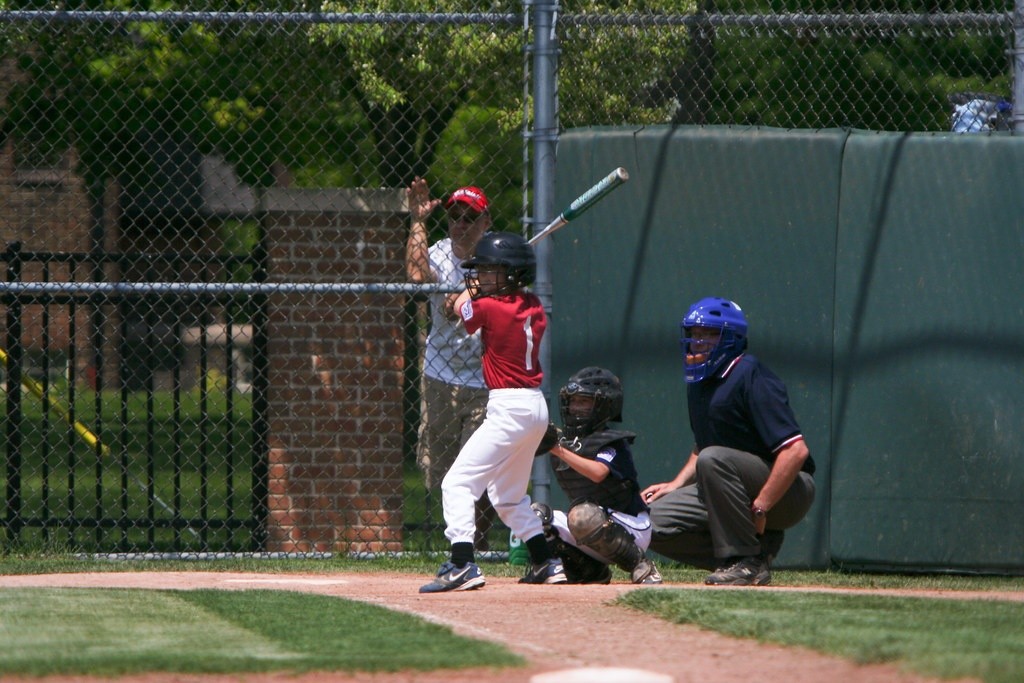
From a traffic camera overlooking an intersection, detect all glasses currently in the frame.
[687,331,723,340]
[448,212,487,223]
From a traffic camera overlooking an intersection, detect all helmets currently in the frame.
[680,297,748,384]
[558,367,623,440]
[461,232,536,301]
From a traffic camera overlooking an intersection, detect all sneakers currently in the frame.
[705,555,771,585]
[518,557,567,584]
[565,565,612,585]
[755,529,784,565]
[419,560,485,593]
[629,559,662,585]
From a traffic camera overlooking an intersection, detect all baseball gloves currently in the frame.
[534,421,558,458]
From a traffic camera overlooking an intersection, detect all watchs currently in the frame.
[751,502,767,519]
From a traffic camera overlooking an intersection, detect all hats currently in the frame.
[444,187,488,213]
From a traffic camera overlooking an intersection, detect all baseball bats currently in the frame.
[527,166,629,246]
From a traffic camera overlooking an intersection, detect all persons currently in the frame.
[473,368,662,585]
[407,177,497,551]
[640,298,816,585]
[420,233,567,593]
